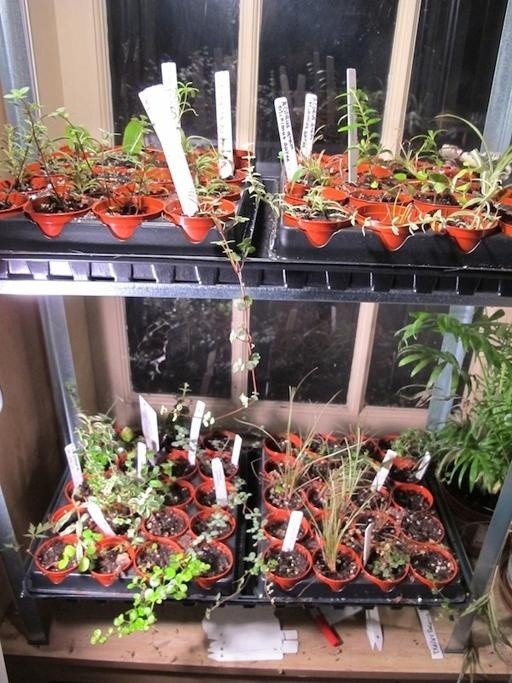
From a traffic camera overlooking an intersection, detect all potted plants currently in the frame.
[260,378,442,596]
[242,86,512,241]
[22,383,244,644]
[0,82,259,424]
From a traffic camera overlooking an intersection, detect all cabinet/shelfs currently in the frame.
[0,0,507,656]
